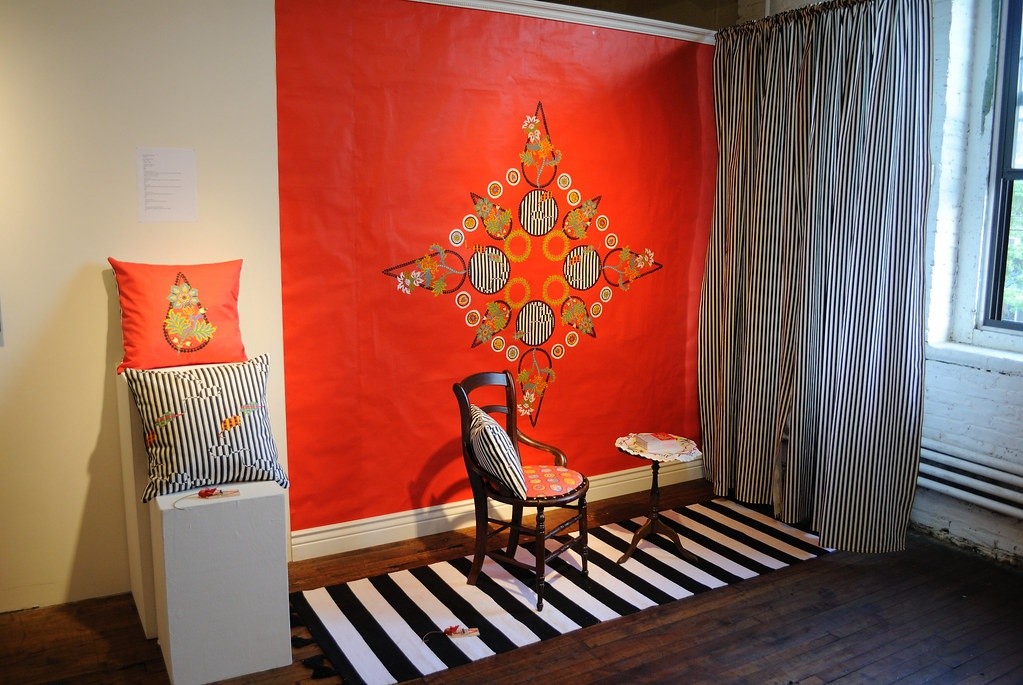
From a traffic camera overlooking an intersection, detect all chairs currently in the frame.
[451,368,591,610]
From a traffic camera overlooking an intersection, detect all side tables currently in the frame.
[613,429,705,568]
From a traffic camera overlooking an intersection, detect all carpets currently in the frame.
[288,492,838,685]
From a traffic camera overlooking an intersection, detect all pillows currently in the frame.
[123,351,291,504]
[107,255,249,375]
[468,403,528,501]
